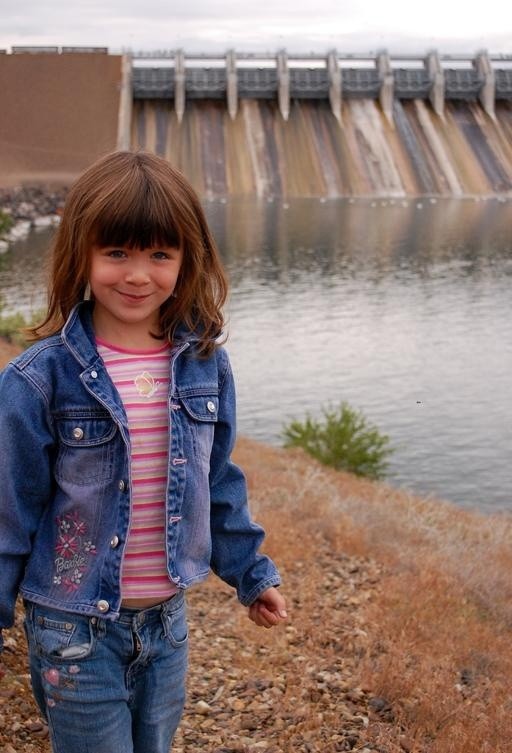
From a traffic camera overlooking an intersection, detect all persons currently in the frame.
[0,149,287,753]
